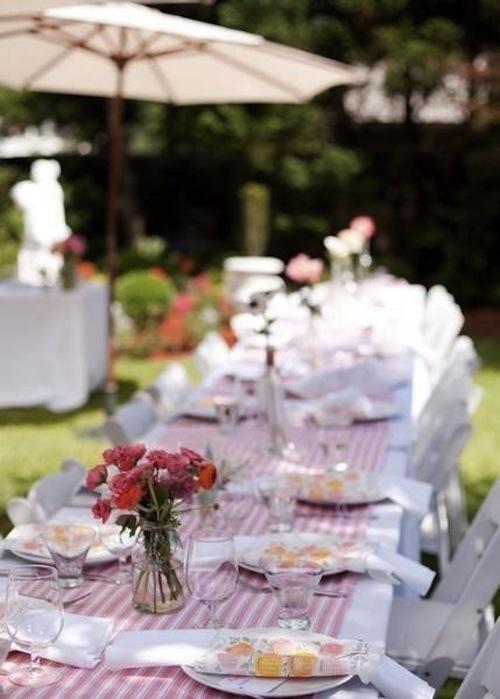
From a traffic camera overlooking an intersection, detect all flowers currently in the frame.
[78,443,224,608]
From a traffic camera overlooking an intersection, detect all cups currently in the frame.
[315,413,353,474]
[184,528,240,629]
[257,475,302,533]
[41,525,97,588]
[212,397,240,435]
[4,565,65,688]
[263,560,326,631]
[98,521,139,582]
[274,408,316,462]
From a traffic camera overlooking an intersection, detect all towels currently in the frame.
[104,626,436,698]
[231,533,436,597]
[8,614,112,668]
[377,471,432,511]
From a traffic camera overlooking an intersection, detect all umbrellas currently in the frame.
[0,0,367,412]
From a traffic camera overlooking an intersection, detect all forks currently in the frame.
[236,578,347,597]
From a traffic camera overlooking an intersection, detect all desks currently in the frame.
[0,277,109,415]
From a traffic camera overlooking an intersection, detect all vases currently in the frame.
[130,524,185,613]
[262,348,287,453]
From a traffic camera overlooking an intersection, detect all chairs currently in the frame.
[100,391,151,450]
[7,458,89,528]
[376,480,498,687]
[151,359,193,411]
[245,295,278,353]
[405,366,482,551]
[447,619,497,699]
[399,394,474,559]
[414,334,478,438]
[198,328,237,374]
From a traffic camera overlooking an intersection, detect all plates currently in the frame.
[298,469,388,504]
[236,533,346,576]
[7,523,131,566]
[180,627,354,697]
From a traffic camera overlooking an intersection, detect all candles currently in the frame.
[2,338,416,698]
